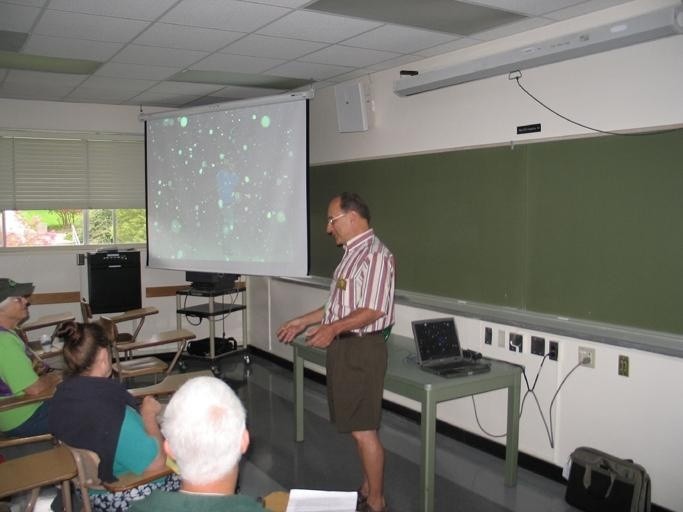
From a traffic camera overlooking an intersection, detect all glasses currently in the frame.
[328,213,346,224]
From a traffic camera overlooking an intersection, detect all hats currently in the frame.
[0,277,33,302]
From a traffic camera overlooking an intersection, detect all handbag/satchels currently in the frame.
[565,448,650,510]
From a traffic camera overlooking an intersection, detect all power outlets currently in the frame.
[549,342,559,360]
[578,346,596,368]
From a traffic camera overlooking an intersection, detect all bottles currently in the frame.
[39,334,51,353]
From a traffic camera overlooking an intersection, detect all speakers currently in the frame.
[86,251,142,314]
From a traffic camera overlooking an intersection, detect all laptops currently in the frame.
[411,317,492,380]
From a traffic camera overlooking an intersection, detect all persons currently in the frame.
[48,319,182,511]
[276,192,397,512]
[0,277,64,438]
[122,375,290,512]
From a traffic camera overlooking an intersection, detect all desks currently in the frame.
[285,321,523,512]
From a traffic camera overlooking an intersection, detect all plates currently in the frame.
[118,356,161,370]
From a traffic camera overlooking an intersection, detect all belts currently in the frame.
[336,330,385,339]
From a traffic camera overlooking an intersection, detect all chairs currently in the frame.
[0,282,290,512]
